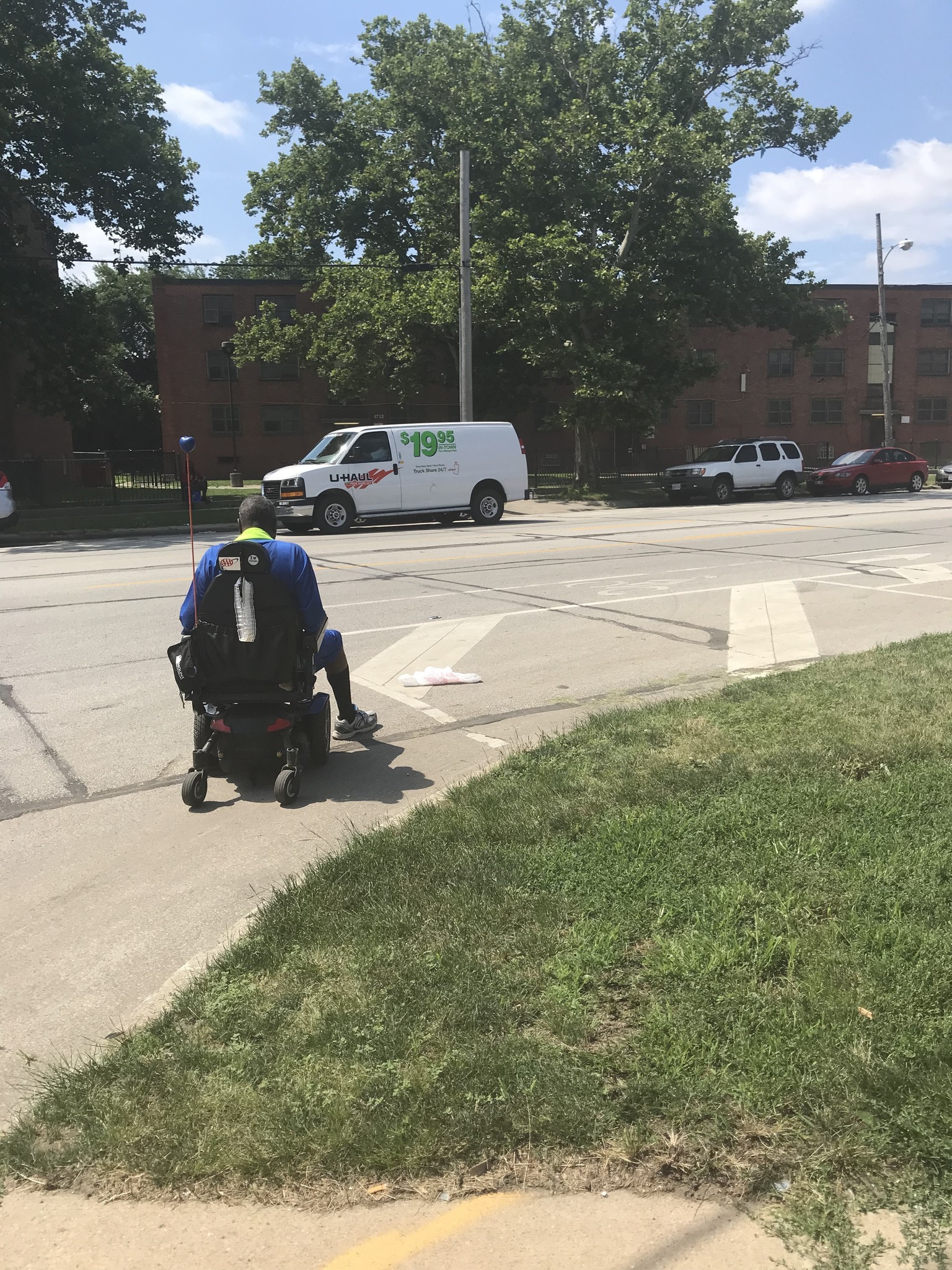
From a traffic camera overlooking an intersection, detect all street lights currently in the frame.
[218,340,245,488]
[876,212,915,448]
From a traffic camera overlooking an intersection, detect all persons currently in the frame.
[180,495,378,740]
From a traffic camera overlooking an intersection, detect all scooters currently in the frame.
[166,540,332,809]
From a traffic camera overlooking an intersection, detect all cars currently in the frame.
[0,469,21,533]
[935,460,952,489]
[806,446,931,497]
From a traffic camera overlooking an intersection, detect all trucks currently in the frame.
[260,421,530,536]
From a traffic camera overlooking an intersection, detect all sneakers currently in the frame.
[332,707,378,740]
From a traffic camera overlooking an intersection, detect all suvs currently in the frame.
[662,436,805,506]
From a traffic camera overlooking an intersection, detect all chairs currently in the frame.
[167,540,326,703]
[876,452,886,460]
[897,454,905,459]
